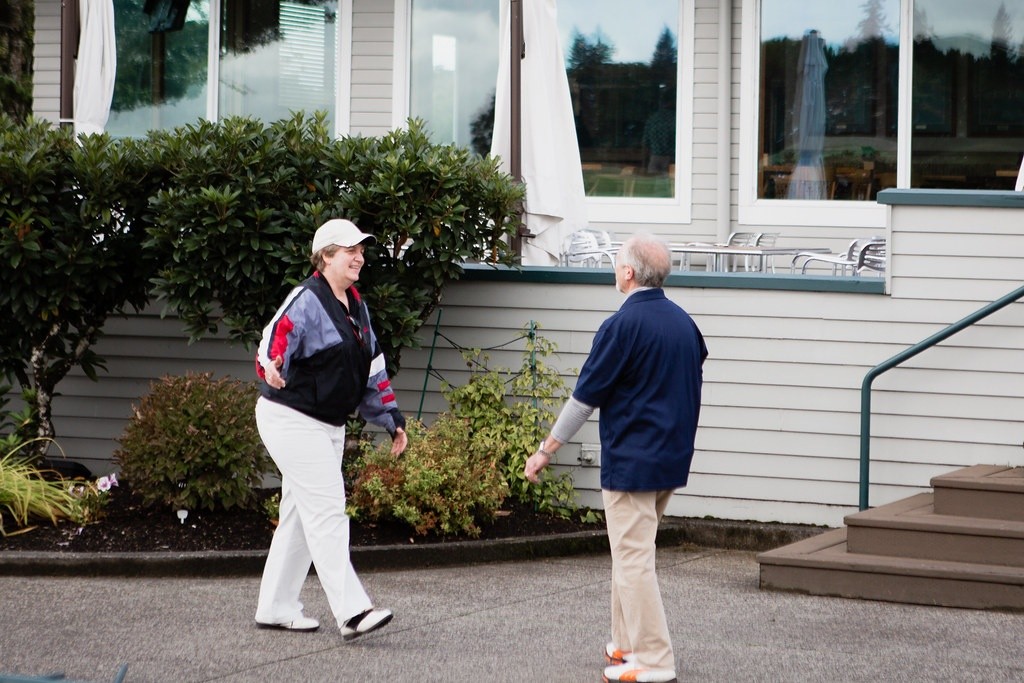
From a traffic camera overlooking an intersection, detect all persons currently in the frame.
[255,219,410,640]
[641,88,675,176]
[523,239,710,683]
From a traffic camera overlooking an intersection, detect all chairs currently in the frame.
[825,166,872,200]
[559,227,886,277]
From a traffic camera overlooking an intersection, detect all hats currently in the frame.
[312,219,377,256]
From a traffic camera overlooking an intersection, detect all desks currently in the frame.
[668,246,832,273]
[763,148,887,201]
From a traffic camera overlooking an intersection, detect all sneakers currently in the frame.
[257,609,320,632]
[341,607,393,642]
[604,657,677,683]
[605,643,631,663]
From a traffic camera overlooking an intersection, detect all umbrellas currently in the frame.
[73,0,117,200]
[488,1,589,267]
[782,29,828,201]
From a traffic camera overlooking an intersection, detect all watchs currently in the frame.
[539,441,554,458]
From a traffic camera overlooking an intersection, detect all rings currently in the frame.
[268,376,271,379]
